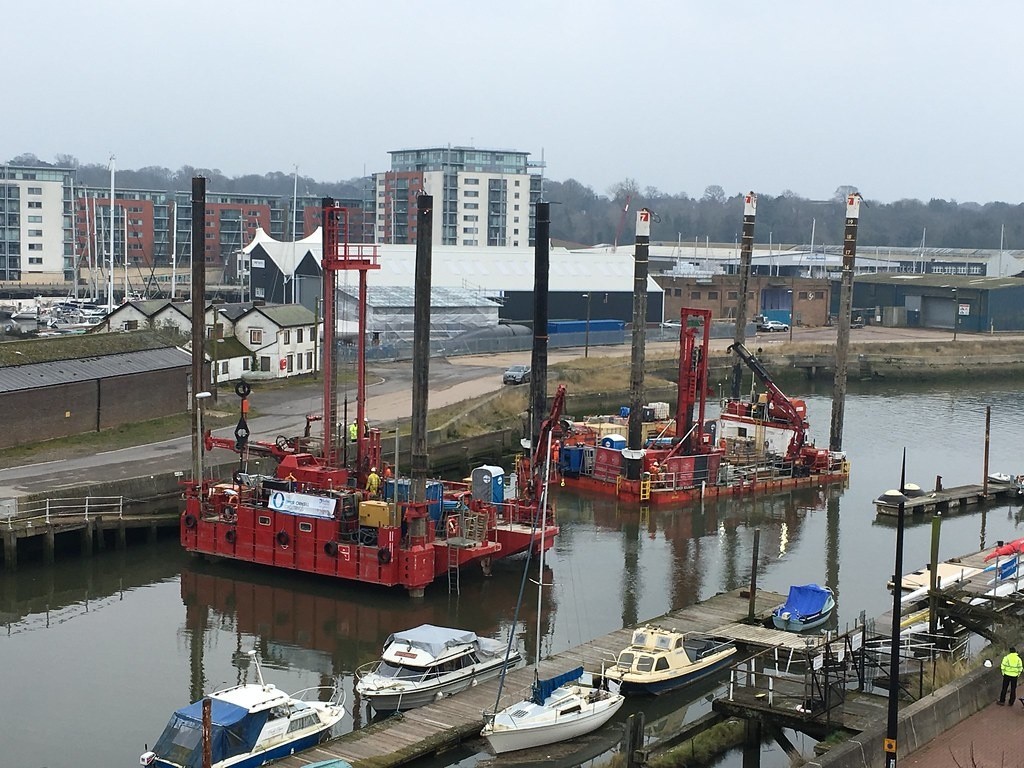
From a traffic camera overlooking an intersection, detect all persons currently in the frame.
[720,437,726,455]
[349,418,369,443]
[996,647,1023,707]
[365,467,381,494]
[649,462,659,489]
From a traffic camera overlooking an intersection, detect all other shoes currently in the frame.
[997,701,1005,705]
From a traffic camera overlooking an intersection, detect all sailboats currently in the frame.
[480,429,627,754]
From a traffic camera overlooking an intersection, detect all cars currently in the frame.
[761,321,789,332]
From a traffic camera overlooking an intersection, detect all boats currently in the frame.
[987,472,1012,484]
[770,583,835,633]
[138,650,347,768]
[603,623,737,698]
[177,176,562,598]
[0,152,250,339]
[546,189,864,509]
[353,623,522,714]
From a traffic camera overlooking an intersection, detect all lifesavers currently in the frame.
[229,495,240,509]
[447,519,459,533]
[523,487,534,501]
